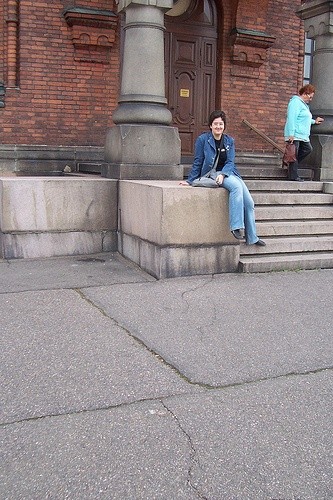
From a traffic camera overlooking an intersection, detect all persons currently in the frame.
[180,110,266,246]
[282,84,324,181]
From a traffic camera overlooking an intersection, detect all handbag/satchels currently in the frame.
[282,140,296,166]
[192,169,219,188]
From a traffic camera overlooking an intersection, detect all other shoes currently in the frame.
[254,240,266,246]
[231,229,243,239]
[290,177,304,181]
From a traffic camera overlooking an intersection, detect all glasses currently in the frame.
[306,93,313,100]
